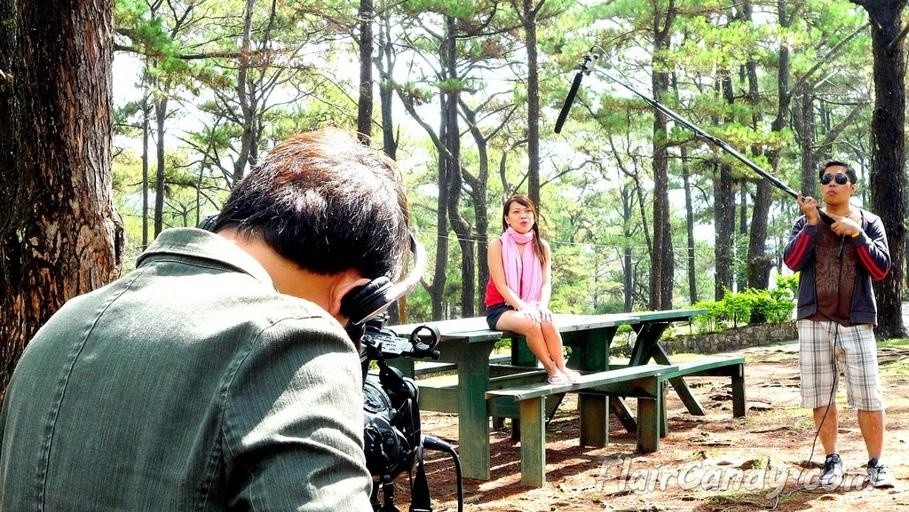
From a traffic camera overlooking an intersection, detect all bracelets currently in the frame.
[852,231,860,238]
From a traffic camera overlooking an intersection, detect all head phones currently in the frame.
[192,211,430,327]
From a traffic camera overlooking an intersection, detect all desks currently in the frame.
[385,307,708,481]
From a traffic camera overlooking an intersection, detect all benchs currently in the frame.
[368,352,746,487]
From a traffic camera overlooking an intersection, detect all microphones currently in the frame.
[550,70,587,133]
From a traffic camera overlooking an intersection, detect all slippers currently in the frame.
[548,371,567,385]
[563,368,583,384]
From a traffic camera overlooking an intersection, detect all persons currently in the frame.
[783,161,896,487]
[484,193,584,387]
[0,122,418,512]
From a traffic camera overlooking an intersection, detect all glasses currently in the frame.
[820,171,850,187]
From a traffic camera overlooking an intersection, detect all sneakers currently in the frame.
[820,453,843,488]
[867,458,895,488]
